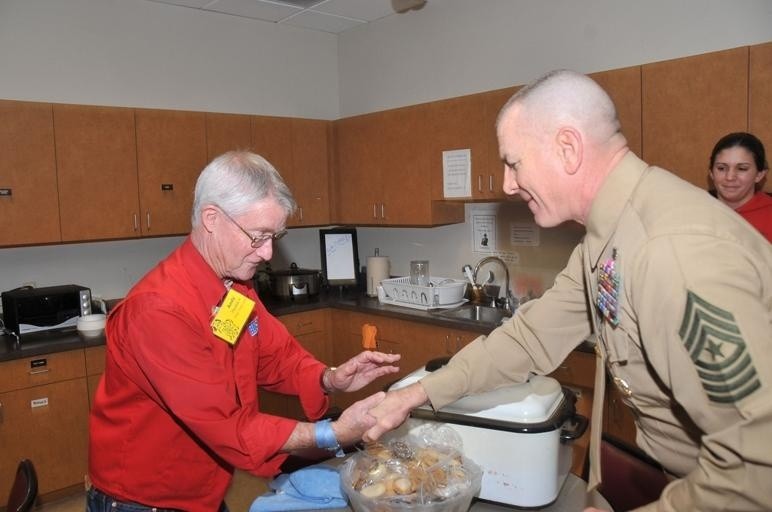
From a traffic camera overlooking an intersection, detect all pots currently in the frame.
[271,263,320,299]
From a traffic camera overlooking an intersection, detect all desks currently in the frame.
[223,446,620,512]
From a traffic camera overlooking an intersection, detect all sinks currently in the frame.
[430,304,513,325]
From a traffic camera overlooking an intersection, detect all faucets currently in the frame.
[473,256,514,310]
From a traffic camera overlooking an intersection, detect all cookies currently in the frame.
[350,440,465,498]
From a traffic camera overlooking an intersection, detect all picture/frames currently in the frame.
[317,227,360,282]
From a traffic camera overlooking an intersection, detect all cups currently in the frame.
[510,296,529,317]
[469,287,484,304]
[410,261,429,286]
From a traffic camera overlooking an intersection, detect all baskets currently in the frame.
[381,276,466,306]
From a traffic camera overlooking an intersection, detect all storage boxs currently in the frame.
[382,354,590,508]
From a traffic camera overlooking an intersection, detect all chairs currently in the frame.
[1,457,42,511]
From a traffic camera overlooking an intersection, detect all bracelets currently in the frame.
[315,418,345,459]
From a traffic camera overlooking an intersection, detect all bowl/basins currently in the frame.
[341,450,483,510]
[77,314,107,337]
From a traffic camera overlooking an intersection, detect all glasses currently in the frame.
[214,204,288,248]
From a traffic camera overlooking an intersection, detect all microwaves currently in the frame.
[2,282,93,335]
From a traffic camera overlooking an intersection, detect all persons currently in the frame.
[361,67,772,512]
[83,149,412,512]
[708,131,772,245]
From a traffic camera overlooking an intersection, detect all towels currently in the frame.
[244,462,351,512]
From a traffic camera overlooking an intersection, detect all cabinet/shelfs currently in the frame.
[207,114,333,229]
[334,102,466,227]
[1,102,63,249]
[259,307,344,423]
[1,345,92,504]
[330,307,407,417]
[401,315,489,382]
[53,102,209,246]
[551,343,609,444]
[608,362,666,471]
[85,344,113,409]
[748,42,770,192]
[589,66,641,163]
[430,87,526,202]
[641,47,747,194]
